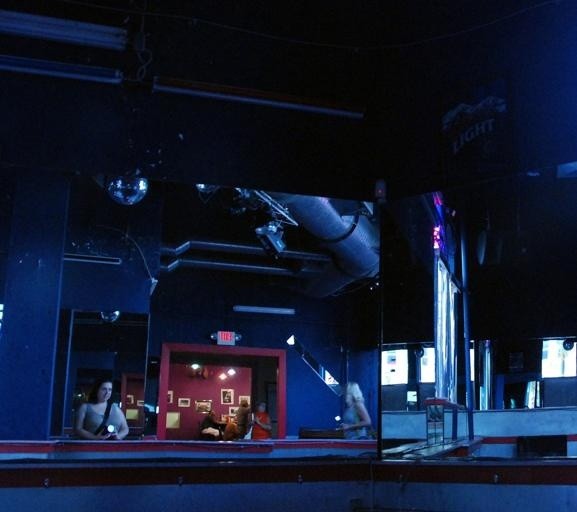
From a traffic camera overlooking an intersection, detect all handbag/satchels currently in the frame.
[244,432,253,440]
[359,432,377,440]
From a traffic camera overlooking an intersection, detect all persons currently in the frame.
[201,409,220,437]
[251,400,273,439]
[236,399,251,439]
[336,381,372,439]
[224,422,241,440]
[74,378,130,440]
